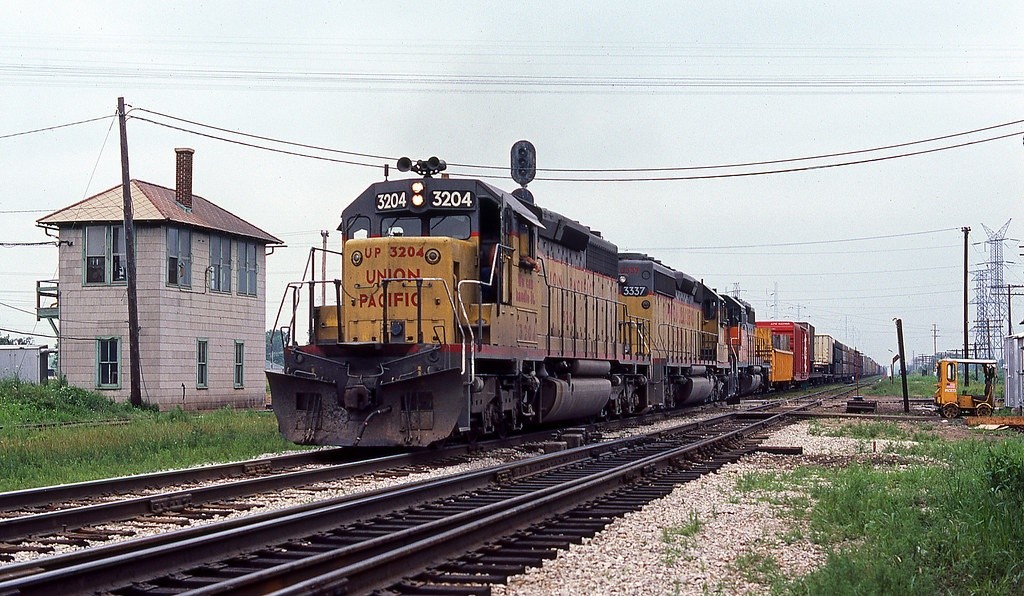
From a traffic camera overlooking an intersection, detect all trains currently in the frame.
[263,141,888,448]
[934,333,1024,419]
